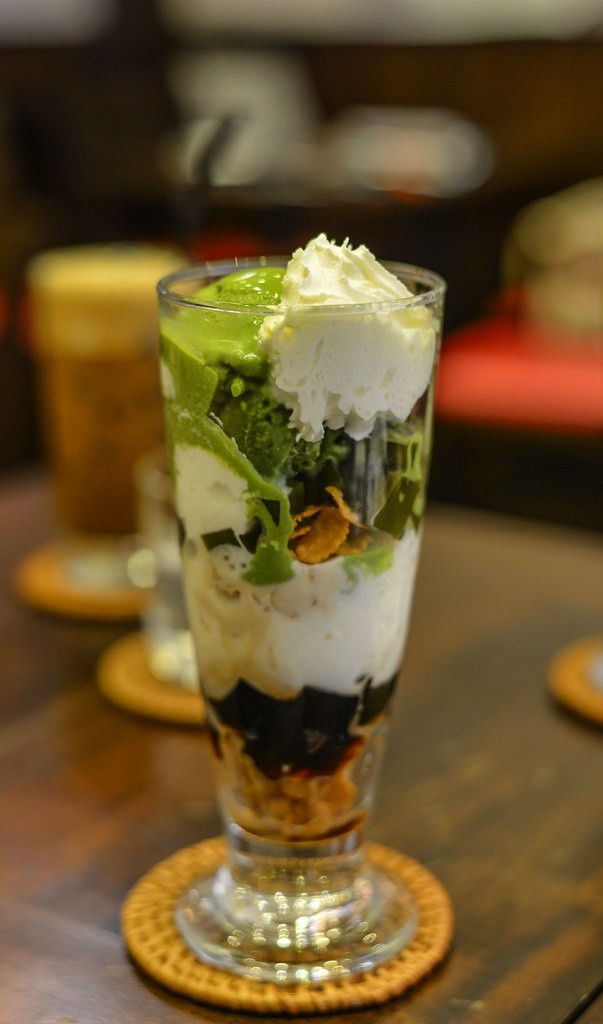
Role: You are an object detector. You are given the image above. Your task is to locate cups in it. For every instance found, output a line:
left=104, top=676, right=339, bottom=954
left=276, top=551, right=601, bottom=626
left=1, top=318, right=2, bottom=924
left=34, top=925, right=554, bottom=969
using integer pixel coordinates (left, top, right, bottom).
left=132, top=443, right=202, bottom=699
left=30, top=247, right=203, bottom=589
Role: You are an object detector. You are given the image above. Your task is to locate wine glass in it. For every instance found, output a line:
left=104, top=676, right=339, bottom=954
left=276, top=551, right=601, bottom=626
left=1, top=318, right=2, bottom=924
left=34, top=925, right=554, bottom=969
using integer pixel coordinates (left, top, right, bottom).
left=157, top=258, right=446, bottom=986
